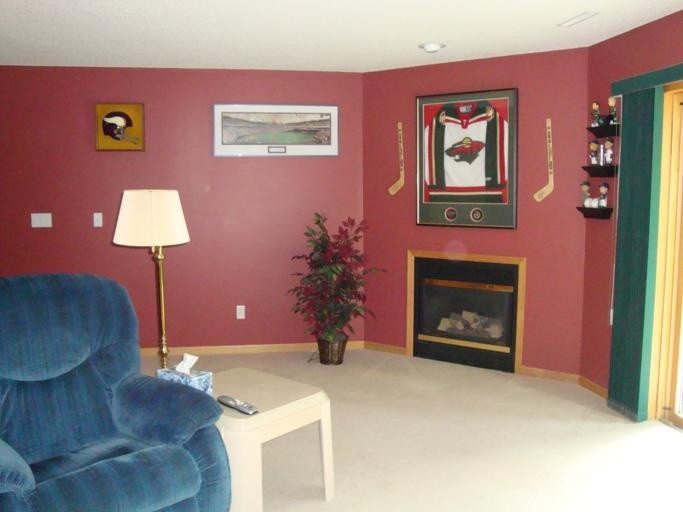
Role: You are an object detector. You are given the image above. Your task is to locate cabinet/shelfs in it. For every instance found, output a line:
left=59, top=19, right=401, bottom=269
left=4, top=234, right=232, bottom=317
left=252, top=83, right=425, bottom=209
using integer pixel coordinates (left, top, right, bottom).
left=572, top=125, right=621, bottom=221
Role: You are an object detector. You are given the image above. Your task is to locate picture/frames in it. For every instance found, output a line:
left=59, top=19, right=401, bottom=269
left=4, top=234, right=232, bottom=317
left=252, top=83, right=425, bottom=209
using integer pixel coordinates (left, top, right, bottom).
left=211, top=100, right=338, bottom=158
left=94, top=101, right=145, bottom=153
left=413, top=87, right=519, bottom=230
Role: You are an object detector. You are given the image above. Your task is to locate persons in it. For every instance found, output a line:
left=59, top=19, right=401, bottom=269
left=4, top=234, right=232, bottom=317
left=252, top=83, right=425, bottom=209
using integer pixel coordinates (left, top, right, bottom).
left=601, top=136, right=613, bottom=166
left=607, top=97, right=617, bottom=124
left=579, top=181, right=592, bottom=208
left=590, top=101, right=600, bottom=127
left=588, top=140, right=599, bottom=165
left=597, top=182, right=609, bottom=208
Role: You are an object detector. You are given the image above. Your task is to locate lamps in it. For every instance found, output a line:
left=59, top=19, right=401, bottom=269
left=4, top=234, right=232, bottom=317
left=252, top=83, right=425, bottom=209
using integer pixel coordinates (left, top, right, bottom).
left=113, top=188, right=190, bottom=368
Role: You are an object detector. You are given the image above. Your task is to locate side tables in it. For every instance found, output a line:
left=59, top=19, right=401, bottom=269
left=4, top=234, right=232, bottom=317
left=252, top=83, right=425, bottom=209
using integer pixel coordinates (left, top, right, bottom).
left=203, top=365, right=336, bottom=512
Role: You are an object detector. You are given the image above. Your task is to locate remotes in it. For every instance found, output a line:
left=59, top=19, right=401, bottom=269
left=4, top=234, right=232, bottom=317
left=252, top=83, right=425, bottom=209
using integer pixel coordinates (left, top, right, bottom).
left=218, top=395, right=259, bottom=415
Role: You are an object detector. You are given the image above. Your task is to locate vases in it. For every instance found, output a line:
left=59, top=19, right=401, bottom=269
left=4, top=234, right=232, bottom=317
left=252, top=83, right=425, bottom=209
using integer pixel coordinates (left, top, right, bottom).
left=315, top=335, right=347, bottom=366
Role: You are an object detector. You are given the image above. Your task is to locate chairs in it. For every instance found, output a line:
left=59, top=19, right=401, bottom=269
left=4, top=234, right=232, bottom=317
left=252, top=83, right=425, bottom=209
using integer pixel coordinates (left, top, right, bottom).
left=0, top=271, right=238, bottom=512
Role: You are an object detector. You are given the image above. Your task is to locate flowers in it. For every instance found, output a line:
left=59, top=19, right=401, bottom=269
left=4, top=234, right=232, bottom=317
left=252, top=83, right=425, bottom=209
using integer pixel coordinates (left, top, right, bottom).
left=284, top=209, right=389, bottom=343
